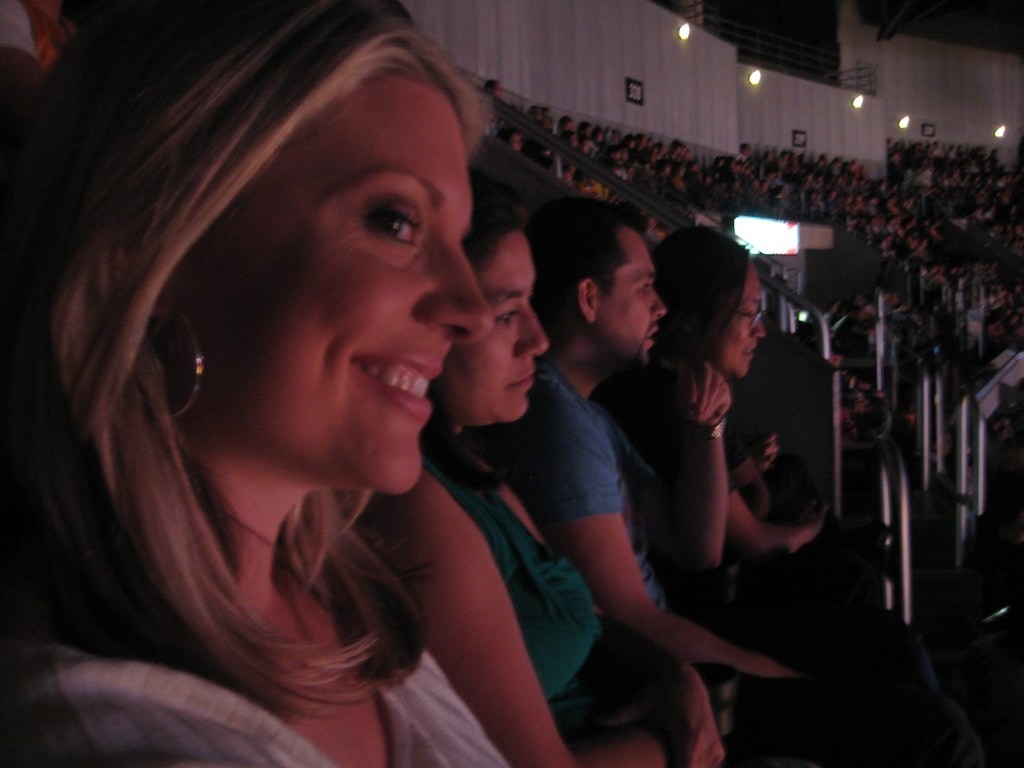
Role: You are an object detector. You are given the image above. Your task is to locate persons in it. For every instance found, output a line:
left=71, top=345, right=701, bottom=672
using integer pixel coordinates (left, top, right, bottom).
left=480, top=71, right=1023, bottom=353
left=1, top=1, right=514, bottom=767
left=357, top=200, right=996, bottom=767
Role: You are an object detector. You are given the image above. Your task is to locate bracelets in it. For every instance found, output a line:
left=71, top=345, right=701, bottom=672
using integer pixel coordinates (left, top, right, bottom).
left=678, top=420, right=728, bottom=442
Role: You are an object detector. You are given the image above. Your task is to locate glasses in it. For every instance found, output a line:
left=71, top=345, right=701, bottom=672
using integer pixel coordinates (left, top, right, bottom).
left=734, top=303, right=768, bottom=326
